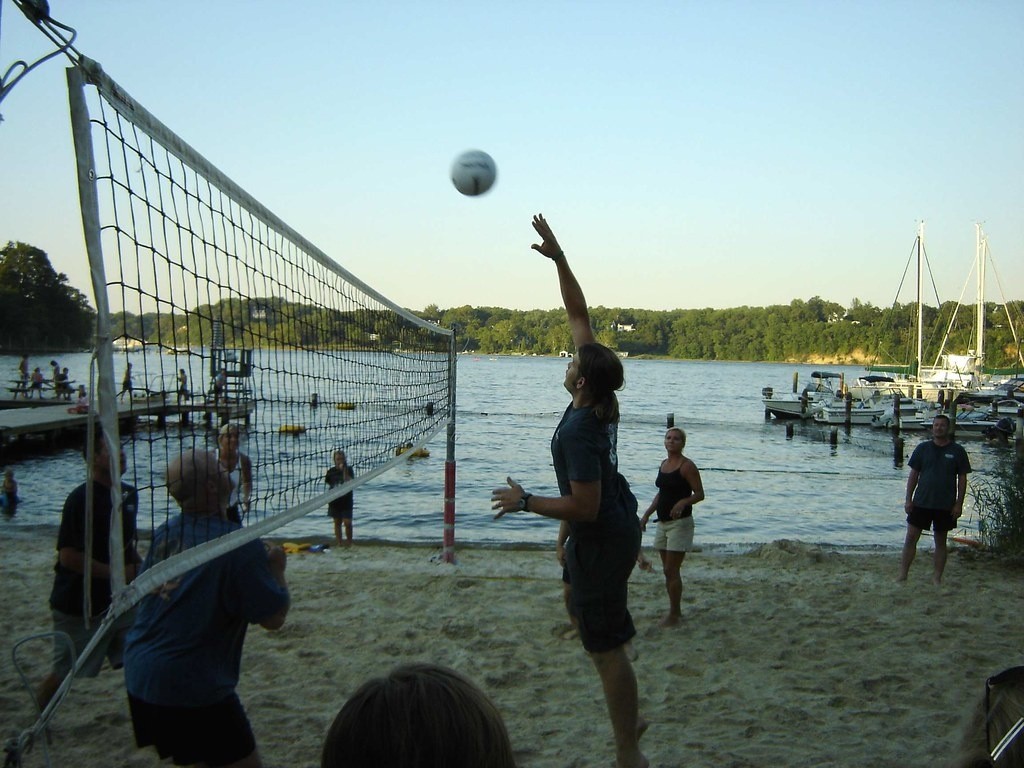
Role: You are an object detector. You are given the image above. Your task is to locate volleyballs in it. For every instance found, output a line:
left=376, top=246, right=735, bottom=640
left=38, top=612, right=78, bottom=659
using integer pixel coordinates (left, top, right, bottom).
left=450, top=147, right=496, bottom=196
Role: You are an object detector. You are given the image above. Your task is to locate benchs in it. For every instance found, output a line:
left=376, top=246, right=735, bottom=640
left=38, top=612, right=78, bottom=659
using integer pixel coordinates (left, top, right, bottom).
left=7, top=387, right=79, bottom=399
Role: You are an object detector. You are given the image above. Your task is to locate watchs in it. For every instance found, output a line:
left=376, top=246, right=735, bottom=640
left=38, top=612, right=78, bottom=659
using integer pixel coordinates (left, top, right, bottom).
left=519, top=492, right=534, bottom=513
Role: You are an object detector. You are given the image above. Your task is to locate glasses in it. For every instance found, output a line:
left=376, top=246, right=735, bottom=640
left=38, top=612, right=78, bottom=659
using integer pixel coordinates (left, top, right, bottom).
left=985, top=665, right=1024, bottom=753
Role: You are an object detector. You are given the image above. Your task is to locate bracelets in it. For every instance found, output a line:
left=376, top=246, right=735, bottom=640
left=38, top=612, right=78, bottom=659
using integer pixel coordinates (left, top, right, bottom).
left=552, top=251, right=564, bottom=261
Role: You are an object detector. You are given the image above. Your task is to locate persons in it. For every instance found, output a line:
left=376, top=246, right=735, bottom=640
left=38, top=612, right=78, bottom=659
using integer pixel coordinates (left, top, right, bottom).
left=206, top=422, right=251, bottom=529
left=1, top=470, right=17, bottom=517
left=640, top=428, right=705, bottom=630
left=124, top=448, right=291, bottom=768
left=324, top=450, right=354, bottom=546
left=491, top=213, right=652, bottom=768
left=29, top=432, right=144, bottom=745
left=938, top=385, right=945, bottom=409
left=16, top=352, right=225, bottom=409
left=320, top=660, right=516, bottom=768
left=893, top=415, right=972, bottom=587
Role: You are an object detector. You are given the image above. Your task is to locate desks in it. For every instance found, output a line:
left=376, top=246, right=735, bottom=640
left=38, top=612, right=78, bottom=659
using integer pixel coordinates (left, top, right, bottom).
left=9, top=380, right=54, bottom=399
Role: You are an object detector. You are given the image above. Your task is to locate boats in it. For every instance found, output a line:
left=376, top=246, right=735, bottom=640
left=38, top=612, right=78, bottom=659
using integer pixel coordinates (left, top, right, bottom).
left=760, top=219, right=1024, bottom=447
